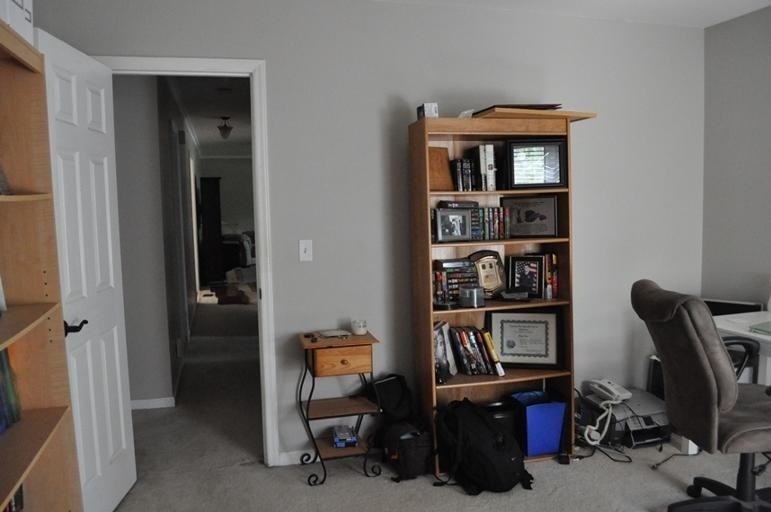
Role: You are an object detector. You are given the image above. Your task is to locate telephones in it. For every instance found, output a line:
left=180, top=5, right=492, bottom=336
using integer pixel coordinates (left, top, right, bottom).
left=590, top=378, right=632, bottom=401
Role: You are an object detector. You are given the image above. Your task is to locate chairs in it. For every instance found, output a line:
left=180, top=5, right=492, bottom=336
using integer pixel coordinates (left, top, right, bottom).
left=239, top=234, right=256, bottom=267
left=630, top=279, right=771, bottom=512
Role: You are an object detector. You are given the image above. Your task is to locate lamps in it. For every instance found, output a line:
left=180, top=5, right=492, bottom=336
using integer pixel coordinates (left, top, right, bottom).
left=217, top=117, right=234, bottom=139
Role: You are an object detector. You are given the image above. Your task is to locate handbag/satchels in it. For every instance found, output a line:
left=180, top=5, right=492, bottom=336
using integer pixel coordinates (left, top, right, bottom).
left=365, top=374, right=414, bottom=424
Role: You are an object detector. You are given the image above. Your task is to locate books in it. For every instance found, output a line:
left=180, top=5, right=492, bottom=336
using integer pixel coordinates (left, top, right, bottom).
left=0, top=276, right=26, bottom=511
left=431, top=200, right=559, bottom=385
left=749, top=320, right=771, bottom=336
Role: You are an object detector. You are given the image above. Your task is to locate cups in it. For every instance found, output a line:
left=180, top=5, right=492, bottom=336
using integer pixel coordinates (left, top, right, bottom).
left=457, top=283, right=487, bottom=309
left=350, top=320, right=368, bottom=336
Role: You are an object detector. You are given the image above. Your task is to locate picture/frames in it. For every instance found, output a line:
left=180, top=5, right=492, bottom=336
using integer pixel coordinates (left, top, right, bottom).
left=484, top=309, right=566, bottom=370
left=505, top=137, right=567, bottom=190
left=499, top=195, right=560, bottom=240
left=433, top=208, right=471, bottom=244
left=508, top=255, right=545, bottom=302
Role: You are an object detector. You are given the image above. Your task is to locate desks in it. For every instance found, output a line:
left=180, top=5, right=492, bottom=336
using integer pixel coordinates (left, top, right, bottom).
left=712, top=311, right=770, bottom=385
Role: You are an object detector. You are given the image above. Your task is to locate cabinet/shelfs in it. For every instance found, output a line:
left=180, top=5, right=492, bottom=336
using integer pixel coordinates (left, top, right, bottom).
left=407, top=117, right=576, bottom=478
left=299, top=328, right=385, bottom=486
left=0, top=19, right=86, bottom=511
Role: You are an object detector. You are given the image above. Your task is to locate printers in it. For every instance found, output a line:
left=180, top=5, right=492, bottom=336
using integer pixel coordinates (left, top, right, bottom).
left=574, top=386, right=669, bottom=448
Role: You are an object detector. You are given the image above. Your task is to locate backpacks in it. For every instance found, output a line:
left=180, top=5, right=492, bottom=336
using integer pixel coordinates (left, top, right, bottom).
left=435, top=398, right=535, bottom=497
left=383, top=417, right=432, bottom=480
